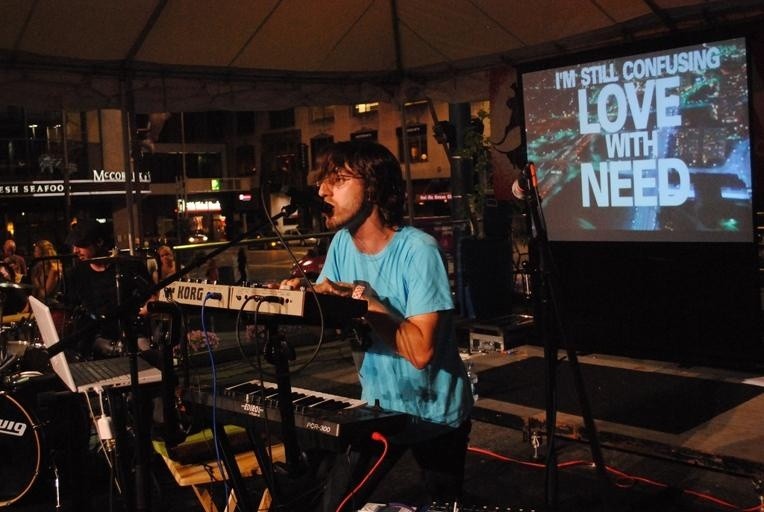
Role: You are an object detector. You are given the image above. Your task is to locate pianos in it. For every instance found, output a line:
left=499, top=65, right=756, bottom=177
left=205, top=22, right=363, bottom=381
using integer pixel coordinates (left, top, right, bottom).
left=181, top=375, right=410, bottom=439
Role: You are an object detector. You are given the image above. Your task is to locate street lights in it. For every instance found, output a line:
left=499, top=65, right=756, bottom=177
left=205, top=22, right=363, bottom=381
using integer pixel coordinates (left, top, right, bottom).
left=29, top=124, right=39, bottom=137
left=53, top=124, right=62, bottom=138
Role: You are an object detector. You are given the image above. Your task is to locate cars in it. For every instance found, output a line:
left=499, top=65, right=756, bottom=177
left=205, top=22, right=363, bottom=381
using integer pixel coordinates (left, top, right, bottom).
left=284, top=229, right=320, bottom=247
left=246, top=229, right=289, bottom=250
left=289, top=225, right=457, bottom=285
left=186, top=233, right=209, bottom=244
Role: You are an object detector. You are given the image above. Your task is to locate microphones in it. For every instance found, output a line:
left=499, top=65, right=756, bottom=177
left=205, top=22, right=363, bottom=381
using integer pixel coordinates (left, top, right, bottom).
left=269, top=175, right=335, bottom=218
left=511, top=162, right=535, bottom=201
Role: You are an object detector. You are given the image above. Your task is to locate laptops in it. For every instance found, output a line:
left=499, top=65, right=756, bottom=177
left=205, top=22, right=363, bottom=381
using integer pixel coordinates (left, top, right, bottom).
left=28, top=294, right=163, bottom=394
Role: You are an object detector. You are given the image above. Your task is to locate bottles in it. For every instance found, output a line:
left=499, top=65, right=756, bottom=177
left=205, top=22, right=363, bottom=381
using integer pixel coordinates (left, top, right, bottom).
left=464, top=361, right=478, bottom=404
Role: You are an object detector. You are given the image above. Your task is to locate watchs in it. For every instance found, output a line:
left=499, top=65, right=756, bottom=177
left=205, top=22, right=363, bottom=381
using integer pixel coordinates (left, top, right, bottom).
left=351, top=280, right=370, bottom=301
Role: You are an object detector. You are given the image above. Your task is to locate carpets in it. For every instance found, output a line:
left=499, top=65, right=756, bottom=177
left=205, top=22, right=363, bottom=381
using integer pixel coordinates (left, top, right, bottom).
left=465, top=354, right=764, bottom=434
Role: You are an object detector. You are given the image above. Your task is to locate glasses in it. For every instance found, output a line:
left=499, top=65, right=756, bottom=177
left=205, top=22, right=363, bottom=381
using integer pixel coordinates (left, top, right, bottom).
left=317, top=175, right=364, bottom=185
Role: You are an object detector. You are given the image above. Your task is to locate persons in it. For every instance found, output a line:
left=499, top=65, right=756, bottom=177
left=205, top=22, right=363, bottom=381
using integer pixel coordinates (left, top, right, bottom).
left=279, top=141, right=474, bottom=512
left=0, top=218, right=219, bottom=359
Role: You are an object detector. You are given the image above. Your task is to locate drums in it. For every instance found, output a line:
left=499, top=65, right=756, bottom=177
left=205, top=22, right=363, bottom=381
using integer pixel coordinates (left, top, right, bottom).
left=0, top=371, right=60, bottom=508
left=0, top=341, right=43, bottom=376
